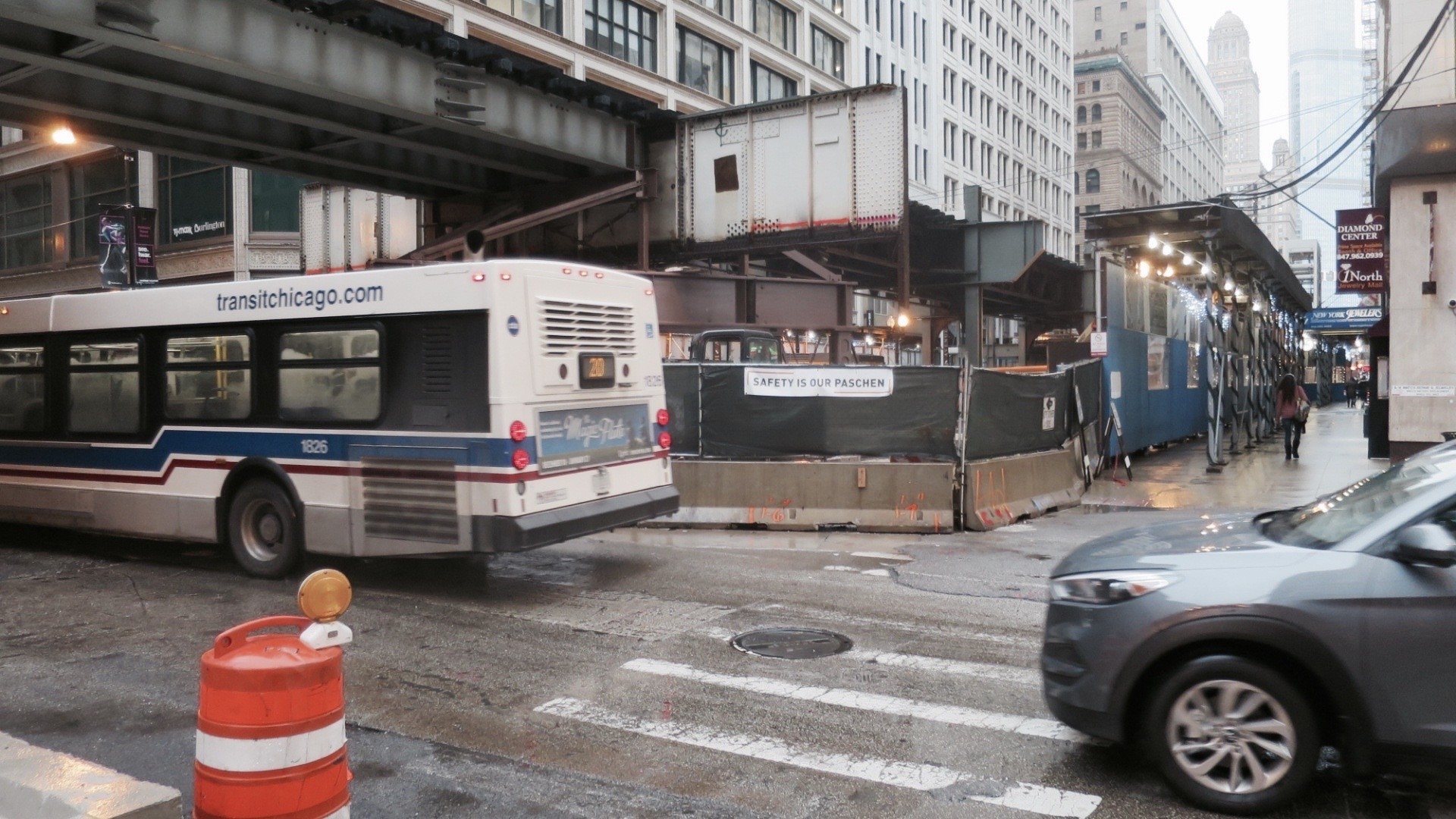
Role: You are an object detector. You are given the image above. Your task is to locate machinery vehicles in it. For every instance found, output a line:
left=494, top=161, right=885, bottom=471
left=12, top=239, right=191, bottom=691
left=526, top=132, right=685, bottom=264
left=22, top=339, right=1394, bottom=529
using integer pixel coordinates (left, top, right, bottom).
left=664, top=328, right=782, bottom=365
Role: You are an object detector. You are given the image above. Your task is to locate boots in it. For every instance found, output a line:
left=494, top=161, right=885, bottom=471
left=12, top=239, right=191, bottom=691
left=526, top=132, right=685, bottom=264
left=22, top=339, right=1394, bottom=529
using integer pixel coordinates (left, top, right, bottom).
left=1283, top=445, right=1290, bottom=459
left=1291, top=439, right=1300, bottom=458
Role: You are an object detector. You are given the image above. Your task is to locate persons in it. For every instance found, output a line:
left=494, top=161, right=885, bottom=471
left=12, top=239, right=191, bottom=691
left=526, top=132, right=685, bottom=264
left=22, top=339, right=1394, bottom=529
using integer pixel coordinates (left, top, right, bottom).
left=1357, top=376, right=1368, bottom=408
left=1274, top=373, right=1310, bottom=459
left=1352, top=378, right=1359, bottom=406
left=1345, top=377, right=1357, bottom=408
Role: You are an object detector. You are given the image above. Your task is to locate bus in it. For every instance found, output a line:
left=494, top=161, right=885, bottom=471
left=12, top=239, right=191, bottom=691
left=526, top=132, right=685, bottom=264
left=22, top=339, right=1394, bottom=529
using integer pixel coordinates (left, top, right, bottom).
left=0, top=257, right=679, bottom=582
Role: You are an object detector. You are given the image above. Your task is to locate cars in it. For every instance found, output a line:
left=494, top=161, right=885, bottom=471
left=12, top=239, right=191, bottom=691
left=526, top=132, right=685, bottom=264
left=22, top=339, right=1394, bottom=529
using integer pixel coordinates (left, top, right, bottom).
left=1038, top=435, right=1456, bottom=814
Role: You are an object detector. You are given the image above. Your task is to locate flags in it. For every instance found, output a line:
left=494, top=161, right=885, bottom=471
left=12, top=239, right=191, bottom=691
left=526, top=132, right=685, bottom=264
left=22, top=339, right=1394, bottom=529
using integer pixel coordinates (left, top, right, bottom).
left=131, top=206, right=158, bottom=284
left=99, top=202, right=131, bottom=289
